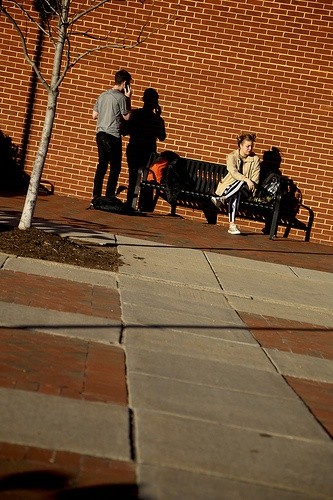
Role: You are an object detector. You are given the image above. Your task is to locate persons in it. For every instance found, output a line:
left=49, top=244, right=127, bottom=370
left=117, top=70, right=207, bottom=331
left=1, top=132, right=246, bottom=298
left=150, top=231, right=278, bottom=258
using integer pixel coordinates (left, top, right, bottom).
left=87, top=68, right=133, bottom=210
left=208, top=131, right=262, bottom=235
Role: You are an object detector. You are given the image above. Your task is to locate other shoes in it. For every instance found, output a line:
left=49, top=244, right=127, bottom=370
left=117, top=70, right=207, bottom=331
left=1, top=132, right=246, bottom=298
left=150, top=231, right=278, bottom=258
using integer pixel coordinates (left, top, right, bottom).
left=103, top=198, right=136, bottom=215
left=90, top=197, right=107, bottom=210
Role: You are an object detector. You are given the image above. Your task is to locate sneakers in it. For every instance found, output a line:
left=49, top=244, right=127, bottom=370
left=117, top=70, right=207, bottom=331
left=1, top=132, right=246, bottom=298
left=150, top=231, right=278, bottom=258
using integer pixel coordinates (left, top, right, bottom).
left=228, top=225, right=241, bottom=234
left=211, top=197, right=226, bottom=211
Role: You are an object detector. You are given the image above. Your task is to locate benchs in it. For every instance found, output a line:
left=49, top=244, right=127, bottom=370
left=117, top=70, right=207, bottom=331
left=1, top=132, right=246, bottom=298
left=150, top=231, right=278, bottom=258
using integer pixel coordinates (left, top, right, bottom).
left=150, top=151, right=283, bottom=241
